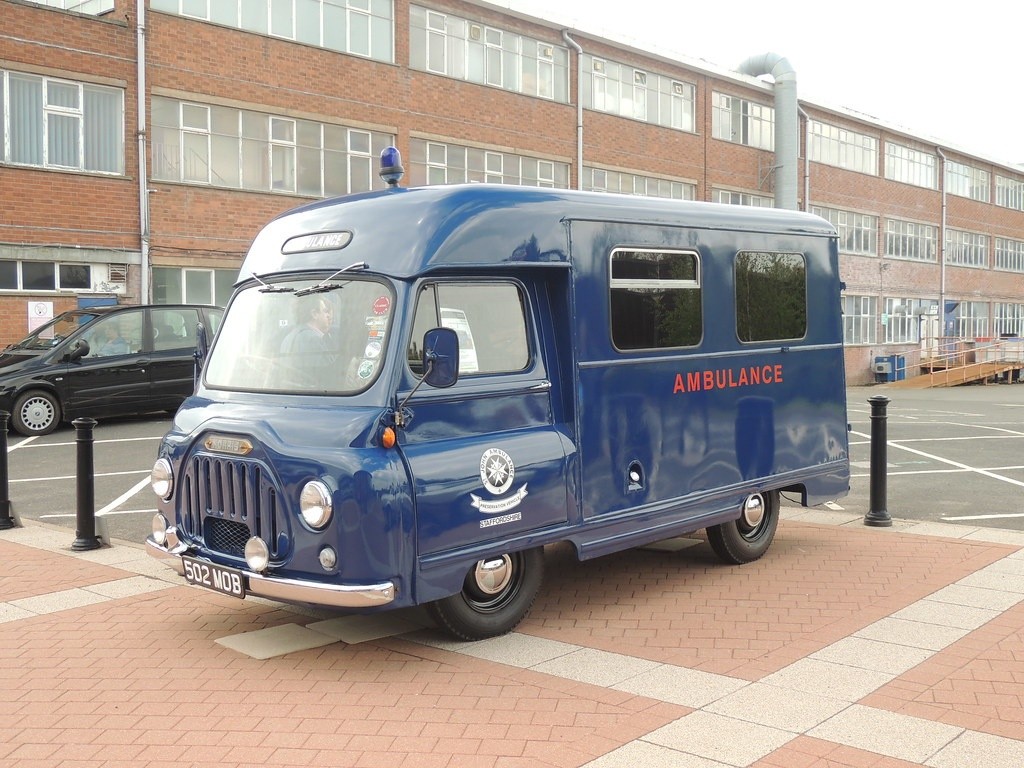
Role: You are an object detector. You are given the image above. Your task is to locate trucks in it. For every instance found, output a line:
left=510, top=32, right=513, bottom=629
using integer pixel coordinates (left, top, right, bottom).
left=145, top=146, right=847, bottom=642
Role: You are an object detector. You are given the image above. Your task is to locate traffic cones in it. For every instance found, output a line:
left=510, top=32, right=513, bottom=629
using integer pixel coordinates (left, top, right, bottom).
left=863, top=395, right=893, bottom=527
left=0, top=405, right=15, bottom=531
left=71, top=415, right=101, bottom=552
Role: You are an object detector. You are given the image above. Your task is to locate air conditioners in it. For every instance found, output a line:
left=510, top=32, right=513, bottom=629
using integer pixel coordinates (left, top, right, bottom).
left=875, top=361, right=892, bottom=373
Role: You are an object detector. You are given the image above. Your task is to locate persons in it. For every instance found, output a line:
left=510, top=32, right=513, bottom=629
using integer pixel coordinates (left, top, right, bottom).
left=93, top=321, right=130, bottom=356
left=283, top=298, right=339, bottom=380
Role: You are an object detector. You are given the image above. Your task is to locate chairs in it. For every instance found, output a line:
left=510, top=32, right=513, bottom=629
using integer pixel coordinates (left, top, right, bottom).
left=159, top=325, right=179, bottom=344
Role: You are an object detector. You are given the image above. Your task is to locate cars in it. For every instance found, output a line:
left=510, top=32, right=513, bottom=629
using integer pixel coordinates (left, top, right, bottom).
left=1, top=308, right=227, bottom=437
